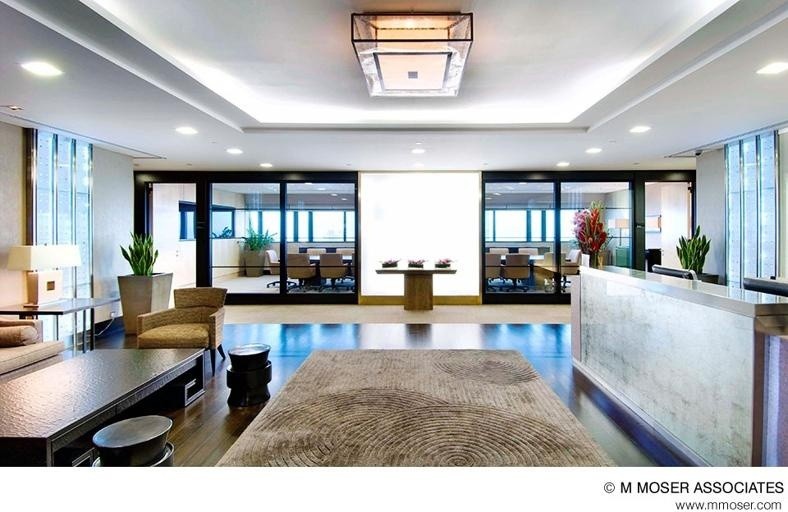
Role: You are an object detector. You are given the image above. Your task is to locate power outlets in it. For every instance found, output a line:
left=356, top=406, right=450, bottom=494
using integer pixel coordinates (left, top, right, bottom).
left=110, top=311, right=115, bottom=319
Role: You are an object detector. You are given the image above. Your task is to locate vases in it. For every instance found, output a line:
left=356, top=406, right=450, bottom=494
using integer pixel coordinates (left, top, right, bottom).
left=434, top=264, right=449, bottom=268
left=381, top=264, right=397, bottom=267
left=582, top=252, right=600, bottom=268
left=407, top=264, right=422, bottom=268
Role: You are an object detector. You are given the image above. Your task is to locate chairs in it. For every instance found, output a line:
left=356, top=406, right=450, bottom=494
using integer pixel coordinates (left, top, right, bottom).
left=518, top=248, right=538, bottom=257
left=286, top=253, right=316, bottom=292
left=306, top=248, right=325, bottom=256
left=533, top=252, right=565, bottom=293
left=264, top=249, right=295, bottom=288
left=350, top=252, right=355, bottom=293
left=318, top=254, right=348, bottom=292
left=485, top=253, right=502, bottom=292
left=562, top=249, right=581, bottom=292
left=489, top=248, right=508, bottom=255
left=335, top=249, right=354, bottom=256
left=499, top=254, right=531, bottom=293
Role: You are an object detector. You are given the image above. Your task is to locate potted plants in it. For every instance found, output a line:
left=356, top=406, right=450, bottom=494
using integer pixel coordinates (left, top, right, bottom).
left=239, top=226, right=276, bottom=277
left=675, top=224, right=720, bottom=285
left=117, top=230, right=174, bottom=337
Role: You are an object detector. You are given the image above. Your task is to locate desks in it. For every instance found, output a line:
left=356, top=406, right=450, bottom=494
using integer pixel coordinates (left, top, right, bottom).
left=499, top=254, right=571, bottom=288
left=375, top=267, right=457, bottom=312
left=0, top=298, right=121, bottom=354
left=644, top=248, right=662, bottom=274
left=277, top=254, right=356, bottom=286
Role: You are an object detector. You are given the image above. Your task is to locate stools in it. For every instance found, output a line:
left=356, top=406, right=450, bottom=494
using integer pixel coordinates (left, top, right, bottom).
left=90, top=415, right=175, bottom=467
left=224, top=342, right=271, bottom=407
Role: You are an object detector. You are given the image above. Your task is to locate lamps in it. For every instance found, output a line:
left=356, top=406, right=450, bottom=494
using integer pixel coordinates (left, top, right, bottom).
left=349, top=12, right=474, bottom=100
left=607, top=217, right=630, bottom=247
left=5, top=244, right=82, bottom=307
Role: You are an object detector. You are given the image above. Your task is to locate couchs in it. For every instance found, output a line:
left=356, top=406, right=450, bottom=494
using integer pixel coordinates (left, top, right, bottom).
left=0, top=317, right=65, bottom=386
left=135, top=286, right=227, bottom=376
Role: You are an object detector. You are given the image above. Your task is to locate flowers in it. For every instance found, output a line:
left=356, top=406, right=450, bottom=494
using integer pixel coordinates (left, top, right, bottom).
left=383, top=258, right=399, bottom=264
left=406, top=259, right=425, bottom=264
left=570, top=198, right=613, bottom=267
left=437, top=259, right=452, bottom=264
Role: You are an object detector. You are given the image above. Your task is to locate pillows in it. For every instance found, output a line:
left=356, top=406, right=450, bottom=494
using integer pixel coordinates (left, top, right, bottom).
left=0, top=326, right=38, bottom=346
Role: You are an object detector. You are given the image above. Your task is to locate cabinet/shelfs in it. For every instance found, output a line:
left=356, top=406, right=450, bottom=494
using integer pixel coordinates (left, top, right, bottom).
left=615, top=246, right=631, bottom=267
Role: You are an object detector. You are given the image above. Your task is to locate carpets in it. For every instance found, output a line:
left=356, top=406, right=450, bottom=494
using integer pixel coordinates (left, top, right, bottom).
left=214, top=347, right=618, bottom=466
